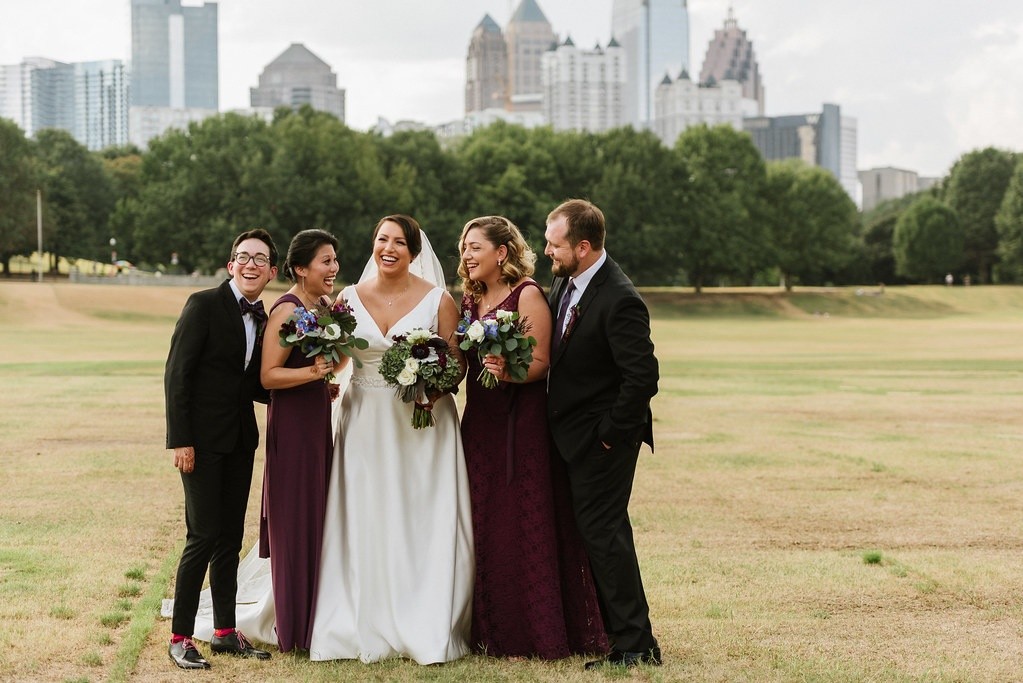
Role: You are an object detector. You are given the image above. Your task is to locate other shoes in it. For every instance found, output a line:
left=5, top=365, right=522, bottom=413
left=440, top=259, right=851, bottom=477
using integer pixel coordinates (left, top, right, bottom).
left=584, top=646, right=661, bottom=670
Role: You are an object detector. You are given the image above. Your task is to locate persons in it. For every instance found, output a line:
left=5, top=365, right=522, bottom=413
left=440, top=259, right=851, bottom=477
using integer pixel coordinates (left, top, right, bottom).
left=543, top=199, right=665, bottom=672
left=455, top=215, right=610, bottom=661
left=259, top=230, right=351, bottom=656
left=322, top=214, right=471, bottom=666
left=163, top=228, right=279, bottom=671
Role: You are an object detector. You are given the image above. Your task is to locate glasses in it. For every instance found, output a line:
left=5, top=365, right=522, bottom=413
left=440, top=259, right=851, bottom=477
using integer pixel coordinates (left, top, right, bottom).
left=234, top=251, right=271, bottom=268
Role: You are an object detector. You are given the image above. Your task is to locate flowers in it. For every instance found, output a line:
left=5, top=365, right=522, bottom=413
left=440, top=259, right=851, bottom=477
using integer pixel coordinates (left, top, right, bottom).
left=279, top=297, right=366, bottom=382
left=457, top=310, right=536, bottom=386
left=377, top=325, right=461, bottom=431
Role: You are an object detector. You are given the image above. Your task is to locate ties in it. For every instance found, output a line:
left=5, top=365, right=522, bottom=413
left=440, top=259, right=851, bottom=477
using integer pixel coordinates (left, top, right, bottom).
left=554, top=277, right=577, bottom=354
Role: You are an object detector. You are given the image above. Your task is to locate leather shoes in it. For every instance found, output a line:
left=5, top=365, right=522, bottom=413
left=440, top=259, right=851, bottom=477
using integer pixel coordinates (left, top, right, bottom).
left=168, top=638, right=211, bottom=669
left=210, top=631, right=272, bottom=660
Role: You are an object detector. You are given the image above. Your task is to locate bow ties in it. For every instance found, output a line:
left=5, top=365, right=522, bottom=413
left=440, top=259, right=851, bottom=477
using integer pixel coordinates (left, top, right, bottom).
left=239, top=297, right=265, bottom=321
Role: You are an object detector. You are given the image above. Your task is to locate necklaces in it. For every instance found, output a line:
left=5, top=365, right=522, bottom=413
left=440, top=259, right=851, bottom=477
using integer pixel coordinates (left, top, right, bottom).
left=481, top=284, right=506, bottom=310
left=375, top=272, right=410, bottom=308
